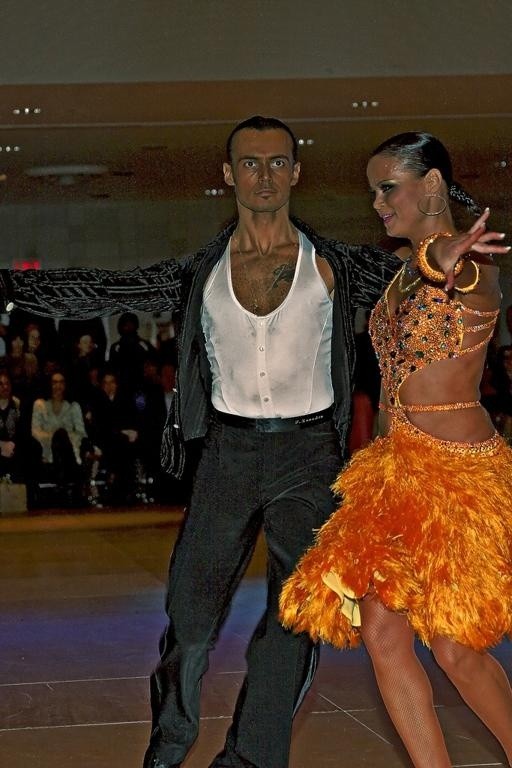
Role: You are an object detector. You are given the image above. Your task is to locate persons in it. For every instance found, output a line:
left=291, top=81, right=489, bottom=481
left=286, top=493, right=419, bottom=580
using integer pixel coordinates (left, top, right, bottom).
left=484, top=345, right=512, bottom=411
left=1, top=115, right=406, bottom=768
left=2, top=310, right=185, bottom=513
left=354, top=309, right=380, bottom=408
left=279, top=131, right=512, bottom=768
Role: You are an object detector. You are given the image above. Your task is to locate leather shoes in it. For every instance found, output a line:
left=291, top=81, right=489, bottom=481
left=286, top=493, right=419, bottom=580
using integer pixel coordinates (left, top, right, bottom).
left=143, top=746, right=181, bottom=768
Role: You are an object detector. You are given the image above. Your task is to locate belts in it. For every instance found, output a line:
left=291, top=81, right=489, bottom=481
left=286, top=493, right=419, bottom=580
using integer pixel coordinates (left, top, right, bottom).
left=216, top=403, right=336, bottom=432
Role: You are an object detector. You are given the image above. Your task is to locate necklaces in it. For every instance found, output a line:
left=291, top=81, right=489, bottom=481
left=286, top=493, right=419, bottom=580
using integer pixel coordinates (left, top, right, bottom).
left=229, top=233, right=299, bottom=313
left=393, top=264, right=423, bottom=292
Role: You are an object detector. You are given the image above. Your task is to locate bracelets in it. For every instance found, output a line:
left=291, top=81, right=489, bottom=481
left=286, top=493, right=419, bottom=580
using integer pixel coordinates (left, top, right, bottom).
left=419, top=231, right=463, bottom=283
left=455, top=257, right=481, bottom=292
